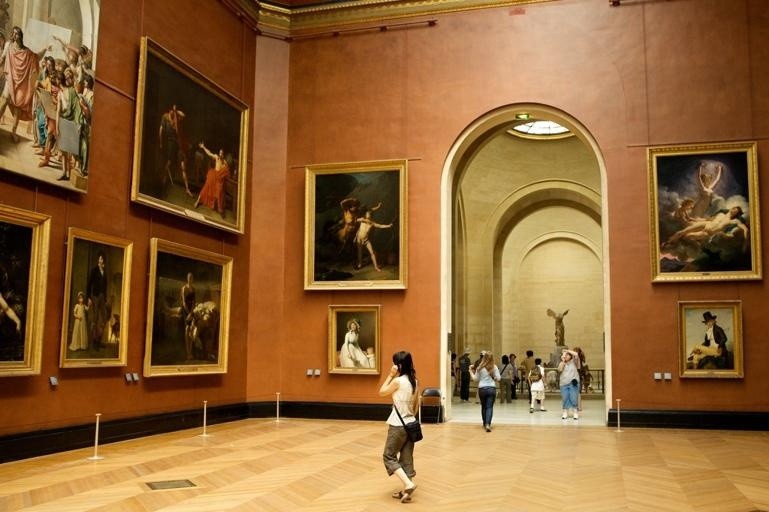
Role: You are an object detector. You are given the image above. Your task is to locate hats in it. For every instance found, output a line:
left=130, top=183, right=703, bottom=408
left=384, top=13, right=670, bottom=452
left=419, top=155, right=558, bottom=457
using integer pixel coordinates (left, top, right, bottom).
left=347, top=318, right=361, bottom=334
left=701, top=311, right=717, bottom=323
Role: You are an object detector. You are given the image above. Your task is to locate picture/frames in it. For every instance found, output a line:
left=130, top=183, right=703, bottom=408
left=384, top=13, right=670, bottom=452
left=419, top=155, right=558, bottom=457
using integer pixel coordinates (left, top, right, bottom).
left=140, top=236, right=234, bottom=380
left=673, top=297, right=745, bottom=383
left=130, top=32, right=251, bottom=239
left=56, top=223, right=135, bottom=372
left=0, top=202, right=55, bottom=381
left=302, top=158, right=412, bottom=293
left=326, top=302, right=384, bottom=376
left=642, top=140, right=767, bottom=287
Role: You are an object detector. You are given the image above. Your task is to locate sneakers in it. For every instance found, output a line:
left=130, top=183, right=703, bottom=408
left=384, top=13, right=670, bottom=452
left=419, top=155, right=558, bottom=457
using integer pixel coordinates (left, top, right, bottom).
left=561, top=413, right=568, bottom=419
left=572, top=411, right=579, bottom=419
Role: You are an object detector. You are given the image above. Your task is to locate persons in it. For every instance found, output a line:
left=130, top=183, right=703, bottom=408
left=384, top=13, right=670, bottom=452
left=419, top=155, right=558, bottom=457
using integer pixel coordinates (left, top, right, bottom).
left=335, top=197, right=383, bottom=261
left=363, top=345, right=376, bottom=368
left=66, top=291, right=92, bottom=351
left=192, top=138, right=232, bottom=219
left=687, top=311, right=732, bottom=369
left=0, top=24, right=92, bottom=188
left=181, top=271, right=197, bottom=360
left=159, top=102, right=194, bottom=199
left=671, top=198, right=703, bottom=224
left=1, top=291, right=23, bottom=332
left=693, top=161, right=725, bottom=215
left=558, top=350, right=581, bottom=419
left=469, top=351, right=501, bottom=432
left=377, top=350, right=422, bottom=503
left=87, top=249, right=110, bottom=344
left=450, top=346, right=593, bottom=405
left=339, top=317, right=370, bottom=367
left=661, top=207, right=748, bottom=257
left=353, top=210, right=392, bottom=273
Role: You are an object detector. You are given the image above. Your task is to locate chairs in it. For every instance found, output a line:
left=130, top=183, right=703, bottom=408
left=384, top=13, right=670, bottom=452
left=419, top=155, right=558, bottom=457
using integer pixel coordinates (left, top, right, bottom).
left=415, top=387, right=443, bottom=425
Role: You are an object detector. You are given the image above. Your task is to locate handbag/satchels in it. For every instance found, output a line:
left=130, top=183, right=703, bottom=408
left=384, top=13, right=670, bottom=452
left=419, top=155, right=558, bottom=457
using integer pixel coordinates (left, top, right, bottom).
left=403, top=421, right=424, bottom=444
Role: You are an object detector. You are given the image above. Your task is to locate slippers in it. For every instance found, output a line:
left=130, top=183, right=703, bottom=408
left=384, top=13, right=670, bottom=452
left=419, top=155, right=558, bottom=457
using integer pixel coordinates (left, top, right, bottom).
left=391, top=480, right=419, bottom=505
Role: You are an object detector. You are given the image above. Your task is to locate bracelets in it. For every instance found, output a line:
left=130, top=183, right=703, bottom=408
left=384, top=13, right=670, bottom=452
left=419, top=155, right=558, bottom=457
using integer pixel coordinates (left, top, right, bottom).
left=388, top=374, right=394, bottom=378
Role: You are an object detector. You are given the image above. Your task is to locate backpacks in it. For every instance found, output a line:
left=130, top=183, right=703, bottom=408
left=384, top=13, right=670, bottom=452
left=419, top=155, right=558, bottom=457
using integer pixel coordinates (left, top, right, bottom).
left=529, top=365, right=542, bottom=383
